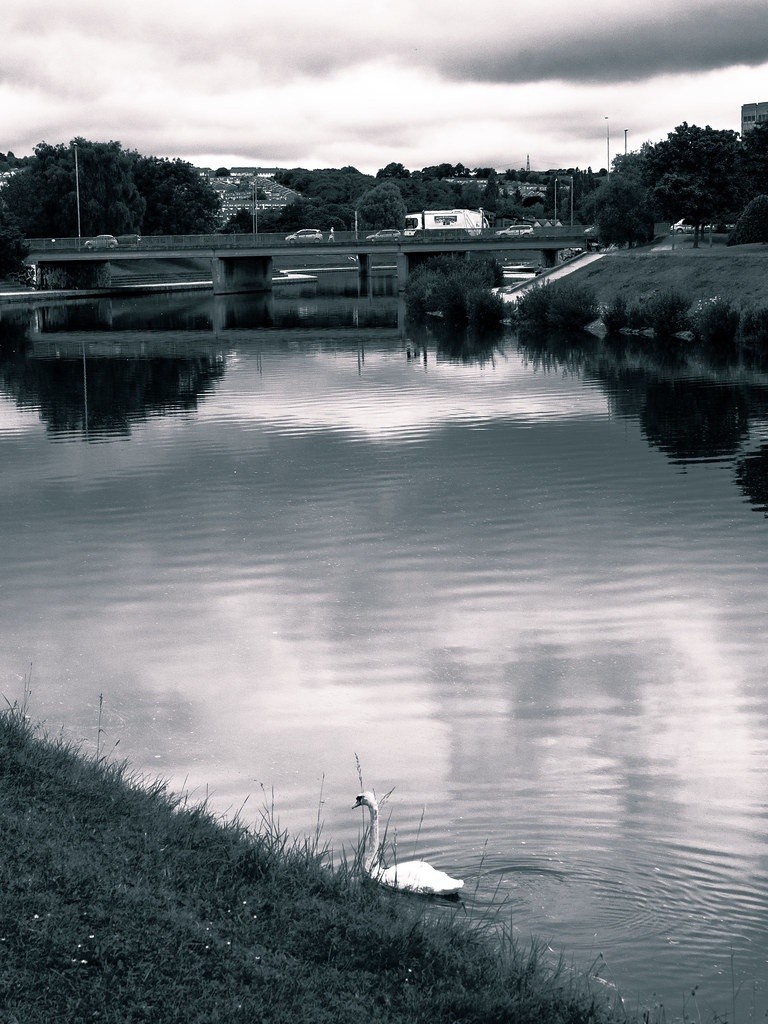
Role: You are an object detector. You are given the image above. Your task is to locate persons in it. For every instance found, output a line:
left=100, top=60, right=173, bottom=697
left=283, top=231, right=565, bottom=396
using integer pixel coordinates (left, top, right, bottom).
left=328, top=227, right=335, bottom=242
left=535, top=264, right=544, bottom=276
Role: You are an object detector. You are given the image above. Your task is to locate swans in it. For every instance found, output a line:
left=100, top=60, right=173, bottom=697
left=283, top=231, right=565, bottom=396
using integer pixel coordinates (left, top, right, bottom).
left=351, top=791, right=465, bottom=897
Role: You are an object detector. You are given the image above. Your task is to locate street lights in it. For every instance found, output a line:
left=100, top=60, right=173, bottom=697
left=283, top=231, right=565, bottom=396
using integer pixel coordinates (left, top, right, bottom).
left=73, top=143, right=81, bottom=252
left=570, top=176, right=573, bottom=227
left=605, top=116, right=610, bottom=183
left=555, top=178, right=558, bottom=226
left=480, top=207, right=484, bottom=237
left=624, top=129, right=628, bottom=155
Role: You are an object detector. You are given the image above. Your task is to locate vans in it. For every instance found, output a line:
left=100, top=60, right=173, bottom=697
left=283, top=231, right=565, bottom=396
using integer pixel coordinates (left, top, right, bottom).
left=670, top=218, right=717, bottom=233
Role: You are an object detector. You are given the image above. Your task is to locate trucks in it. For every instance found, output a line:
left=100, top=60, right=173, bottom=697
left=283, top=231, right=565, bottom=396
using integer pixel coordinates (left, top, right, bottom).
left=404, top=209, right=481, bottom=237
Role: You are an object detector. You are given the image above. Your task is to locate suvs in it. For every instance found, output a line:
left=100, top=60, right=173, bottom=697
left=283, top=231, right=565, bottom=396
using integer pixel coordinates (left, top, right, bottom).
left=285, top=229, right=323, bottom=243
left=496, top=225, right=534, bottom=239
left=366, top=229, right=401, bottom=242
left=85, top=235, right=118, bottom=249
